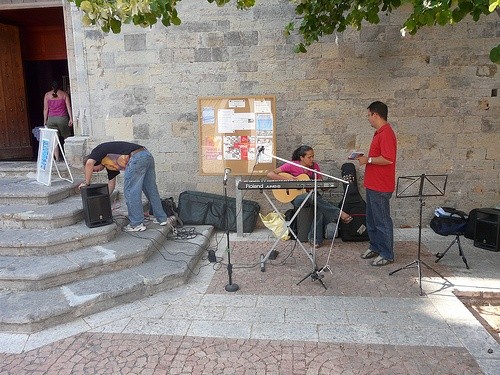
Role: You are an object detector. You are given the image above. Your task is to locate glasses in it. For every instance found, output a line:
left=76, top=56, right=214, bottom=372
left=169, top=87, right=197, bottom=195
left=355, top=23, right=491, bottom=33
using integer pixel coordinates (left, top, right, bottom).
left=366, top=113, right=374, bottom=118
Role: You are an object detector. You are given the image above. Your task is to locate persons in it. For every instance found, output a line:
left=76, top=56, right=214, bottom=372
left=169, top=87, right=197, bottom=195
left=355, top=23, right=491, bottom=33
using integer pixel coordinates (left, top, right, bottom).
left=78, top=141, right=168, bottom=232
left=354, top=102, right=397, bottom=266
left=266, top=144, right=353, bottom=247
left=42, top=72, right=73, bottom=161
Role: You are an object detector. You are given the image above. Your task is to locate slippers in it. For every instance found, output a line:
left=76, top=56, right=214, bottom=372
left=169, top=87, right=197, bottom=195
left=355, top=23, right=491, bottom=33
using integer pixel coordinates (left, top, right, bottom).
left=341, top=216, right=354, bottom=224
left=309, top=240, right=320, bottom=248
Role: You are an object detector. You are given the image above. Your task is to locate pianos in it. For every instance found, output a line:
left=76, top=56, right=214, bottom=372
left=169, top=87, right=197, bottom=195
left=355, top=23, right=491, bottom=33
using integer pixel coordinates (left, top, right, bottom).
left=236, top=178, right=340, bottom=273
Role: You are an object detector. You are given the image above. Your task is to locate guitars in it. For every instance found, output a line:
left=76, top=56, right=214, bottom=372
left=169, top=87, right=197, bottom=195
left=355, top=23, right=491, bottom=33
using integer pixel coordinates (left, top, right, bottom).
left=271, top=172, right=356, bottom=204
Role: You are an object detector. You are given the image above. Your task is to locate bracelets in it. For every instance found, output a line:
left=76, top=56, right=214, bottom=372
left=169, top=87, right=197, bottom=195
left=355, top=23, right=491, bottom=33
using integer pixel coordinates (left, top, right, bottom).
left=84, top=181, right=91, bottom=186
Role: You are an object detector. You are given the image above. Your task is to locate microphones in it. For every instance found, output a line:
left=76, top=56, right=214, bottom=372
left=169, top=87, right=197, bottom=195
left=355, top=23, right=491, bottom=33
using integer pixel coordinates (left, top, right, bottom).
left=223, top=167, right=231, bottom=184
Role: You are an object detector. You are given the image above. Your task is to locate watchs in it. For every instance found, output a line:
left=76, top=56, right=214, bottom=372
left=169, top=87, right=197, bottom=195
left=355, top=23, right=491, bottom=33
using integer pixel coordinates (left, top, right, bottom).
left=368, top=156, right=372, bottom=164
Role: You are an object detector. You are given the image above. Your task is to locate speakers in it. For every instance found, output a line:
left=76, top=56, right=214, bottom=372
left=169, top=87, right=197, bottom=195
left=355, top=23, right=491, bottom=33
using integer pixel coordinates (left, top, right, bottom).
left=473, top=208, right=500, bottom=252
left=80, top=183, right=114, bottom=229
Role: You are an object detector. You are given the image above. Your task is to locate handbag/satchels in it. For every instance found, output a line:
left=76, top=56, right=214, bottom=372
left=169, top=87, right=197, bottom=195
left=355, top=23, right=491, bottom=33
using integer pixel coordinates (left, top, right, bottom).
left=430, top=207, right=468, bottom=236
left=259, top=209, right=291, bottom=241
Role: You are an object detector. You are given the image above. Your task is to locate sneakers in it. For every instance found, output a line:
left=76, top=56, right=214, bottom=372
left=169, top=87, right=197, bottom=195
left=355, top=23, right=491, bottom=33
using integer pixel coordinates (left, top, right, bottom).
left=360, top=249, right=379, bottom=259
left=121, top=223, right=147, bottom=232
left=370, top=255, right=394, bottom=266
left=153, top=218, right=168, bottom=226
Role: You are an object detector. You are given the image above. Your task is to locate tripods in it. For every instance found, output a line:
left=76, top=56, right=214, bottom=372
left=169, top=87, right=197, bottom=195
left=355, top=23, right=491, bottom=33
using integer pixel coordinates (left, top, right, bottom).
left=387, top=174, right=453, bottom=296
